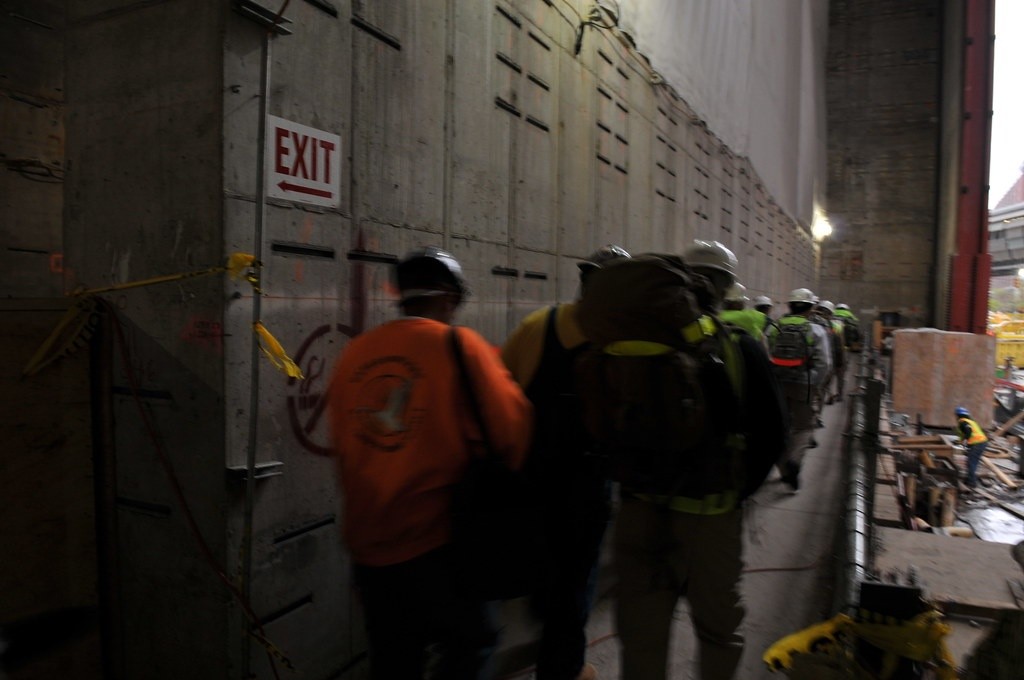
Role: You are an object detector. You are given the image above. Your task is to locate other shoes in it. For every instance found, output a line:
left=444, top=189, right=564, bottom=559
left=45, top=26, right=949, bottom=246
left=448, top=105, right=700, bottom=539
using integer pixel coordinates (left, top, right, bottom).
left=781, top=461, right=799, bottom=490
left=965, top=478, right=976, bottom=488
left=815, top=417, right=823, bottom=429
left=575, top=662, right=597, bottom=680
left=828, top=395, right=834, bottom=404
left=808, top=436, right=817, bottom=449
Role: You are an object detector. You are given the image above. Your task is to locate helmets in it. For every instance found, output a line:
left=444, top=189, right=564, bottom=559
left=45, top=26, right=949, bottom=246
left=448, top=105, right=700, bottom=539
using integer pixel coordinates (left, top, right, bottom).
left=682, top=241, right=737, bottom=279
left=835, top=304, right=850, bottom=310
left=785, top=289, right=820, bottom=304
left=722, top=283, right=750, bottom=302
left=754, top=296, right=772, bottom=308
left=396, top=245, right=466, bottom=299
left=814, top=300, right=836, bottom=315
left=577, top=243, right=632, bottom=272
left=955, top=407, right=970, bottom=415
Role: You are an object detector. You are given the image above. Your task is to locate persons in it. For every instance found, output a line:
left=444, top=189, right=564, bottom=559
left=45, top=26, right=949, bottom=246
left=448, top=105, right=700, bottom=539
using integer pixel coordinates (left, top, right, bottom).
left=501, top=245, right=630, bottom=680
left=726, top=282, right=860, bottom=492
left=954, top=406, right=987, bottom=489
left=327, top=247, right=533, bottom=680
left=611, top=240, right=785, bottom=680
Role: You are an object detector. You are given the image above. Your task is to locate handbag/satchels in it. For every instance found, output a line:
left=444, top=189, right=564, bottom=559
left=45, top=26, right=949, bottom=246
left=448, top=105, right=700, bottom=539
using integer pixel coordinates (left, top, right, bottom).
left=443, top=461, right=537, bottom=598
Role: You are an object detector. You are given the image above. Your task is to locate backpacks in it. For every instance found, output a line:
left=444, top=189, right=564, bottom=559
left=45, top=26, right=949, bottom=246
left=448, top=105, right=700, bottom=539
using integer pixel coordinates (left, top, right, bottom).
left=518, top=307, right=609, bottom=568
left=770, top=321, right=814, bottom=379
left=574, top=252, right=731, bottom=500
